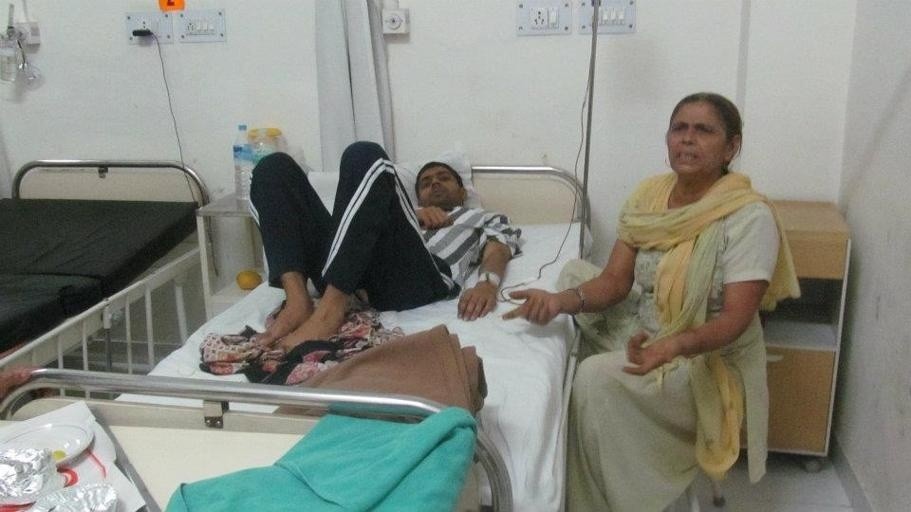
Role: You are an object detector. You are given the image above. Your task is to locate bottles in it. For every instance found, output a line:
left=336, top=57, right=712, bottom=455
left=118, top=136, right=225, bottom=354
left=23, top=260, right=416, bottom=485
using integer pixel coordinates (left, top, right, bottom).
left=233, top=124, right=283, bottom=200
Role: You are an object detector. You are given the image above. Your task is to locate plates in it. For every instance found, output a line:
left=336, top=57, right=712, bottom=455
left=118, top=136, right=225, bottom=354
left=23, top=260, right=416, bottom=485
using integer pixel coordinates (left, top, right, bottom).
left=1, top=422, right=94, bottom=466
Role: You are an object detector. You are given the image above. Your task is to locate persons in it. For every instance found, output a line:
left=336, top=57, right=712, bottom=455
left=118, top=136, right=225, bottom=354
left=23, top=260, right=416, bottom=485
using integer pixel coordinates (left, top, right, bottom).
left=248, top=142, right=525, bottom=352
left=497, top=90, right=788, bottom=509
left=1, top=361, right=37, bottom=404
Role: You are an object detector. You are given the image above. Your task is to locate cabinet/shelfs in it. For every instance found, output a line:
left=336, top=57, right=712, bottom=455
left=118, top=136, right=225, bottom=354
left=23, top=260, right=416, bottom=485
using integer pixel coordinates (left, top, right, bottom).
left=740, top=233, right=851, bottom=456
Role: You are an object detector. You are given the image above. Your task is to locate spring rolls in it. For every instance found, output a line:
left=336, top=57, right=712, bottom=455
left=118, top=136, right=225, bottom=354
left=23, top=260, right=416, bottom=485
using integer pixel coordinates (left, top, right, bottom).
left=77, top=482, right=118, bottom=511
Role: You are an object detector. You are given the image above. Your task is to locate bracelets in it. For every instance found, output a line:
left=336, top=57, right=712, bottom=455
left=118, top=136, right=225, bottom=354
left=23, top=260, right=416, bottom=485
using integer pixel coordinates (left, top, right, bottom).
left=572, top=285, right=588, bottom=314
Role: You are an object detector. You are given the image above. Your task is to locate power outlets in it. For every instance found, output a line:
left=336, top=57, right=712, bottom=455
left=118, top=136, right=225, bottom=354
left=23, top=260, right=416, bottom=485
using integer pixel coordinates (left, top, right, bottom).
left=517, top=0, right=573, bottom=35
left=126, top=12, right=173, bottom=44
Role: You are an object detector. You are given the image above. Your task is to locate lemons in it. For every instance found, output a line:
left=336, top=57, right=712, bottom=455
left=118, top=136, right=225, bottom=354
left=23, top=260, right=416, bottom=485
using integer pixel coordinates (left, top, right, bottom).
left=237, top=270, right=262, bottom=290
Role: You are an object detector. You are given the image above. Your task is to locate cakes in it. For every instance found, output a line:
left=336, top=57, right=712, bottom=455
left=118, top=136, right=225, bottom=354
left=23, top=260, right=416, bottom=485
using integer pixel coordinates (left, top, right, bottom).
left=1, top=444, right=56, bottom=506
